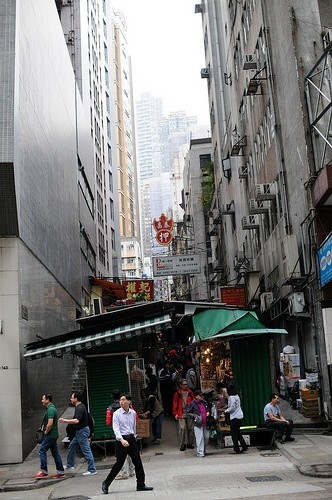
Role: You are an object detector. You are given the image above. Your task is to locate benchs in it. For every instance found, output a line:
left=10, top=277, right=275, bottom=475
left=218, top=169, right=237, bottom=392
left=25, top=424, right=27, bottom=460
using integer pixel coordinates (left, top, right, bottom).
left=216, top=427, right=277, bottom=447
left=90, top=438, right=143, bottom=457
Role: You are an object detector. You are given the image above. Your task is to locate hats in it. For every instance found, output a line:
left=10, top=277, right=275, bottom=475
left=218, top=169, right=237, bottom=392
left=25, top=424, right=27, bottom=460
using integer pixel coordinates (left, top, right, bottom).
left=194, top=390, right=204, bottom=396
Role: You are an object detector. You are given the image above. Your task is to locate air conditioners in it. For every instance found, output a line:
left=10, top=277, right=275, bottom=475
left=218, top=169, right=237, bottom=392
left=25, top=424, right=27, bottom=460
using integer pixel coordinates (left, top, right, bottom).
left=234, top=251, right=244, bottom=269
left=255, top=183, right=275, bottom=200
left=213, top=260, right=221, bottom=271
left=245, top=69, right=258, bottom=93
left=195, top=4, right=202, bottom=13
left=93, top=297, right=104, bottom=314
left=209, top=209, right=220, bottom=232
left=323, top=29, right=332, bottom=51
left=288, top=292, right=305, bottom=313
left=250, top=199, right=258, bottom=209
left=260, top=292, right=273, bottom=311
left=238, top=166, right=248, bottom=178
left=243, top=54, right=256, bottom=69
left=201, top=68, right=209, bottom=78
left=241, top=215, right=259, bottom=228
left=221, top=204, right=234, bottom=215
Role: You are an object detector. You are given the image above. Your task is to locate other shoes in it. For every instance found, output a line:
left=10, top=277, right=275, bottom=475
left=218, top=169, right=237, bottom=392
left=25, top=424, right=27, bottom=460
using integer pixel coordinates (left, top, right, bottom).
left=286, top=437, right=298, bottom=441
left=102, top=482, right=108, bottom=494
left=63, top=466, right=74, bottom=472
left=51, top=473, right=65, bottom=479
left=137, top=485, right=153, bottom=491
left=180, top=444, right=186, bottom=451
left=115, top=476, right=129, bottom=481
left=82, top=471, right=97, bottom=476
left=196, top=451, right=207, bottom=457
left=279, top=439, right=284, bottom=444
left=241, top=448, right=249, bottom=453
left=229, top=450, right=242, bottom=454
left=187, top=444, right=194, bottom=449
left=35, top=471, right=48, bottom=479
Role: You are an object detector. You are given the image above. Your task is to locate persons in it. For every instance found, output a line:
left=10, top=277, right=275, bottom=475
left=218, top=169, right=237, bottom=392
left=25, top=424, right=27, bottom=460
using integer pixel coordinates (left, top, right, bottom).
left=217, top=385, right=249, bottom=454
left=151, top=337, right=194, bottom=419
left=106, top=389, right=139, bottom=480
left=263, top=393, right=295, bottom=444
left=34, top=393, right=65, bottom=479
left=59, top=391, right=97, bottom=476
left=172, top=379, right=195, bottom=451
left=140, top=389, right=164, bottom=445
left=102, top=393, right=153, bottom=493
left=186, top=390, right=210, bottom=458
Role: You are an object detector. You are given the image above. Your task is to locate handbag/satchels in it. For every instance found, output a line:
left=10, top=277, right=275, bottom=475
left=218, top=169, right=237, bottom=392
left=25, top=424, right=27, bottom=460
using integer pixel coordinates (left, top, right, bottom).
left=35, top=430, right=44, bottom=444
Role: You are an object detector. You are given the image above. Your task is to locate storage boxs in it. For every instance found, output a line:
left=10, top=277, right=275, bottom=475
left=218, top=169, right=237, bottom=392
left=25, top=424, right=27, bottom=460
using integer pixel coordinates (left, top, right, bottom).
left=137, top=419, right=152, bottom=438
left=225, top=435, right=250, bottom=447
left=279, top=352, right=320, bottom=417
